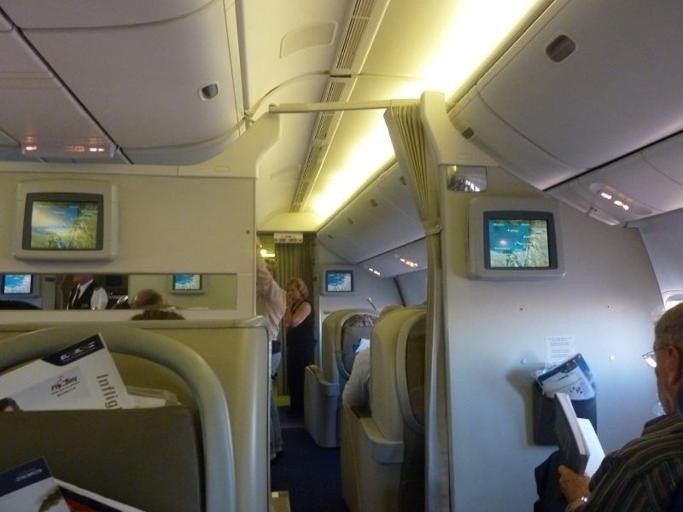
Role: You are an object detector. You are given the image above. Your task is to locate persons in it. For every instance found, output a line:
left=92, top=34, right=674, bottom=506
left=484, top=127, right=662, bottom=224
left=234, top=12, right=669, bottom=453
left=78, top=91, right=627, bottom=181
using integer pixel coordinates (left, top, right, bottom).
left=111, top=289, right=185, bottom=320
left=284, top=277, right=314, bottom=419
left=256, top=235, right=288, bottom=460
left=558, top=302, right=683, bottom=512
left=342, top=305, right=404, bottom=408
left=66, top=273, right=109, bottom=310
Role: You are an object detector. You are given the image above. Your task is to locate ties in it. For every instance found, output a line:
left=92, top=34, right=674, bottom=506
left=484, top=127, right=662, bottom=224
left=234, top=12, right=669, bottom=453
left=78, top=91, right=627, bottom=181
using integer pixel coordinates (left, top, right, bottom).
left=71, top=285, right=81, bottom=305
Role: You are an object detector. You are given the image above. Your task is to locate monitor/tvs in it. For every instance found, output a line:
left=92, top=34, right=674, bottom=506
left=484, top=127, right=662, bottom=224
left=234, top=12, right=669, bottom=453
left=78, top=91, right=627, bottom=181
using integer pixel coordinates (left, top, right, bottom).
left=325, top=269, right=354, bottom=292
left=13, top=181, right=116, bottom=259
left=468, top=196, right=569, bottom=278
left=172, top=274, right=206, bottom=292
left=2, top=275, right=39, bottom=294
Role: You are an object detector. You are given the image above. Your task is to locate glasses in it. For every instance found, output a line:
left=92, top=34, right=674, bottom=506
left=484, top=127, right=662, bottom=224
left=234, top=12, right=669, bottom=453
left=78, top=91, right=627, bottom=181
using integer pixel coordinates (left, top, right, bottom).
left=641, top=345, right=670, bottom=369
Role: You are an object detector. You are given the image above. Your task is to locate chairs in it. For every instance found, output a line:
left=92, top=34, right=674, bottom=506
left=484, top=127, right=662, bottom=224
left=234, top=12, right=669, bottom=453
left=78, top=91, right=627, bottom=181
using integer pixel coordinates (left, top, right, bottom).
left=306, top=302, right=431, bottom=511
left=0, top=298, right=292, bottom=512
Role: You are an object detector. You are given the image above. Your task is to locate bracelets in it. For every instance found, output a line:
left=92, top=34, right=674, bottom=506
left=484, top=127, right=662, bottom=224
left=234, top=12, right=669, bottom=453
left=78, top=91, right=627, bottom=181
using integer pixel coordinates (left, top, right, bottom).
left=565, top=496, right=588, bottom=512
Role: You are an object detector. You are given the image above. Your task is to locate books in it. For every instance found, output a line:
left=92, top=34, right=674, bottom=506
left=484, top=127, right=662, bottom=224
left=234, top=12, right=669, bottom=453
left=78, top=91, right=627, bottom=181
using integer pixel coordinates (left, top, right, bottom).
left=552, top=392, right=606, bottom=479
left=54, top=478, right=146, bottom=512
left=126, top=385, right=183, bottom=409
left=0, top=331, right=179, bottom=412
left=0, top=456, right=71, bottom=512
